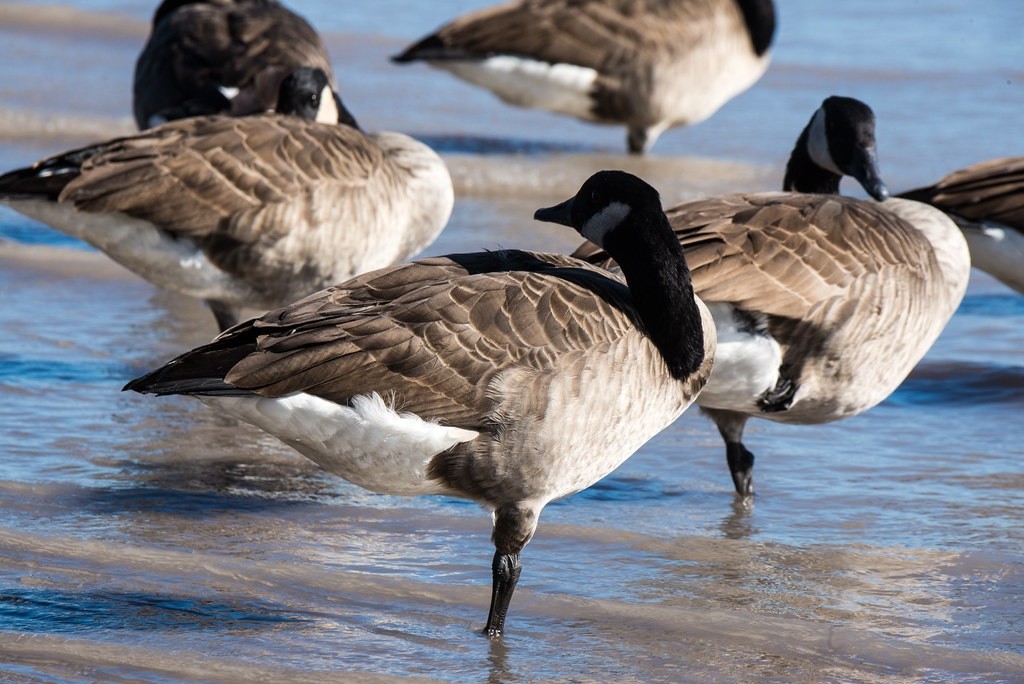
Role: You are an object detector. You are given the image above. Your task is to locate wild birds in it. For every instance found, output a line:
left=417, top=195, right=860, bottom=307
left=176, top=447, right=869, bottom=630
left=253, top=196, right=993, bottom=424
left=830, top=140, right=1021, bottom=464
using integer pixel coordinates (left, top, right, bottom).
left=132, top=1, right=324, bottom=135
left=892, top=158, right=1024, bottom=298
left=568, top=93, right=973, bottom=497
left=120, top=168, right=717, bottom=638
left=386, top=1, right=779, bottom=156
left=1, top=66, right=455, bottom=333
